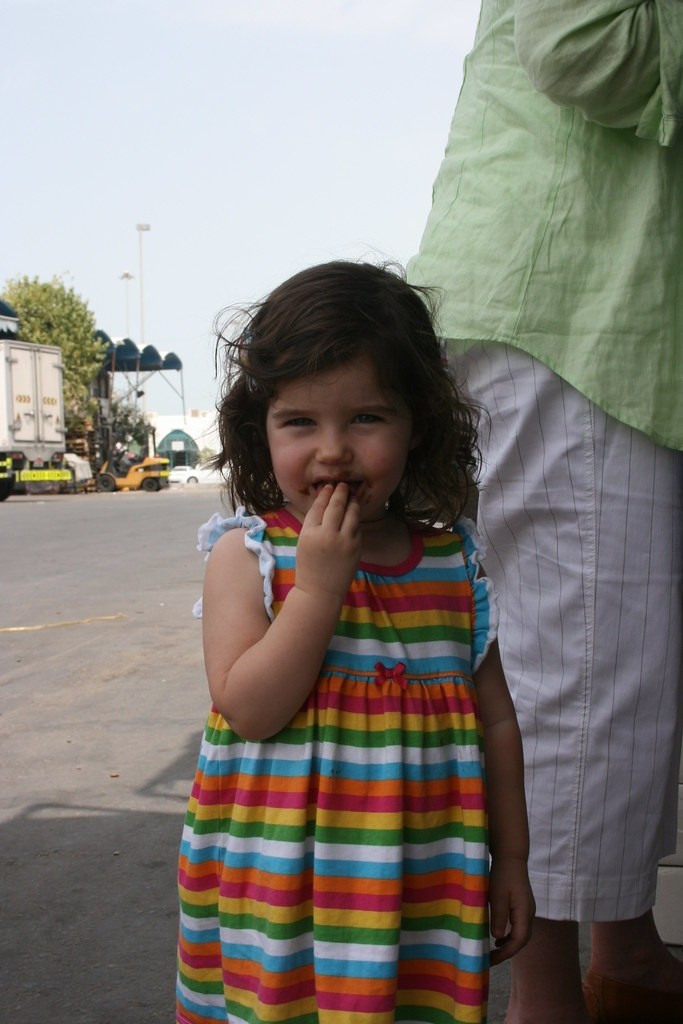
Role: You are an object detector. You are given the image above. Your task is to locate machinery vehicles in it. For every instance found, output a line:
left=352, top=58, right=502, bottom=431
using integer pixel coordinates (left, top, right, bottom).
left=80, top=422, right=170, bottom=492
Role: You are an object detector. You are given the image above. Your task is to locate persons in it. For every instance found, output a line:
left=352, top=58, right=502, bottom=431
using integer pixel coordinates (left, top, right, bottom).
left=172, top=260, right=537, bottom=1024
left=119, top=434, right=140, bottom=468
left=408, top=0, right=683, bottom=1024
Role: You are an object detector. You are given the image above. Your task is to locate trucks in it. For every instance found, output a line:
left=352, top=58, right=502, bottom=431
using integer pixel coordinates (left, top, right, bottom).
left=0, top=339, right=72, bottom=502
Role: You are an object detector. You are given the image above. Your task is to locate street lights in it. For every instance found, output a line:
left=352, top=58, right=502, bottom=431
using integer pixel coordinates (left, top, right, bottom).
left=119, top=272, right=135, bottom=339
left=136, top=223, right=151, bottom=414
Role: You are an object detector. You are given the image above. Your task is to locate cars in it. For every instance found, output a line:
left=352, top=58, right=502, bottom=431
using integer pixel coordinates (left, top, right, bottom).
left=180, top=459, right=234, bottom=485
left=169, top=466, right=192, bottom=481
